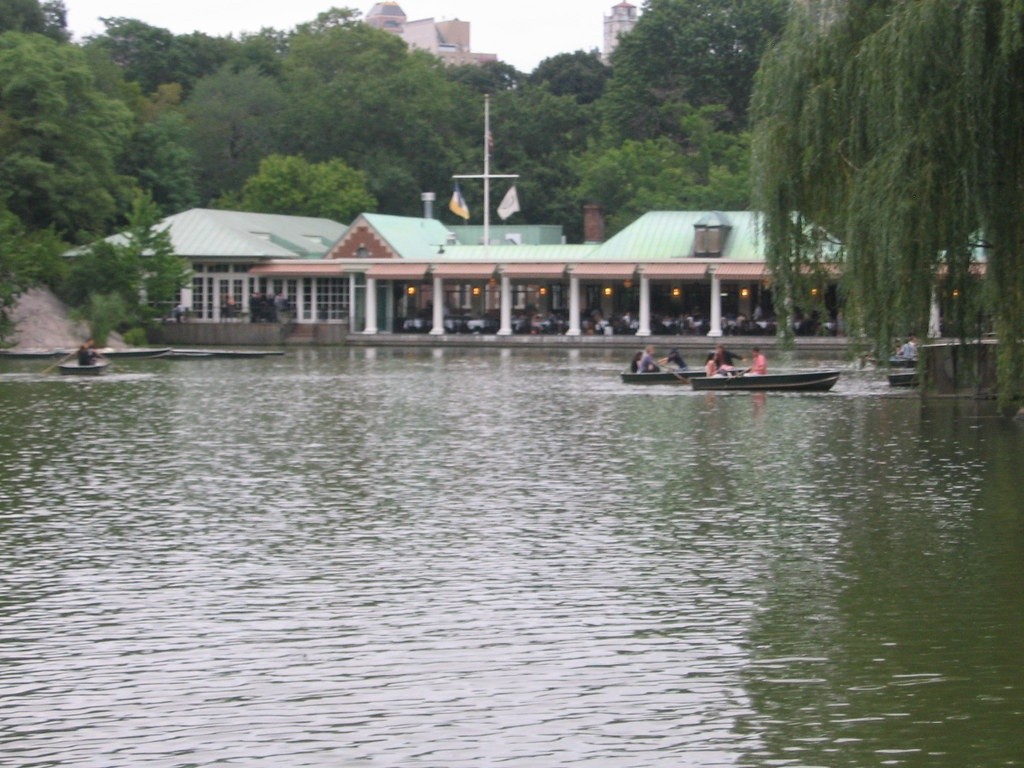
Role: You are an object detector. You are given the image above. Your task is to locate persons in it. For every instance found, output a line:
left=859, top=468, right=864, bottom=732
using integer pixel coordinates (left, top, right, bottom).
left=896, top=333, right=919, bottom=359
left=630, top=344, right=688, bottom=374
left=175, top=293, right=278, bottom=323
left=459, top=304, right=837, bottom=335
left=705, top=343, right=768, bottom=377
left=77, top=339, right=97, bottom=366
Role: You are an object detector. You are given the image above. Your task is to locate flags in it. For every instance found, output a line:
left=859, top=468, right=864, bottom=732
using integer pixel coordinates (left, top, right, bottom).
left=448, top=180, right=471, bottom=220
left=482, top=102, right=493, bottom=156
left=497, top=185, right=521, bottom=221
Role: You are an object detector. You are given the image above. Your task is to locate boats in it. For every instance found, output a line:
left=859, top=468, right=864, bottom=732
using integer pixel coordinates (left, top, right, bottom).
left=57, top=356, right=112, bottom=376
left=619, top=362, right=747, bottom=384
left=686, top=368, right=844, bottom=392
left=886, top=368, right=920, bottom=387
left=859, top=352, right=920, bottom=367
